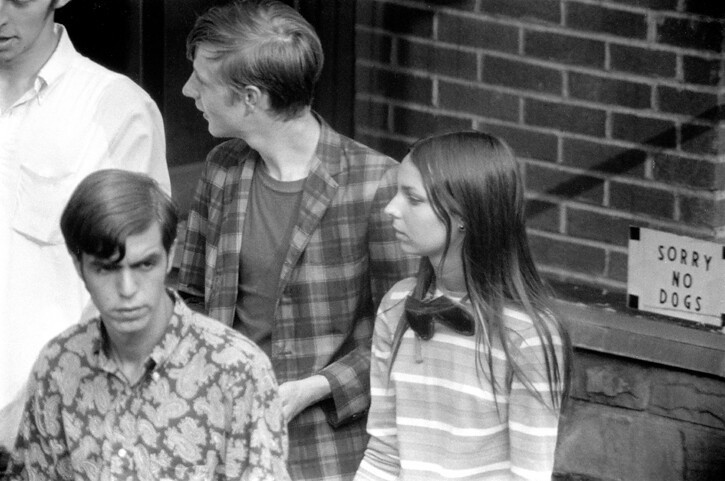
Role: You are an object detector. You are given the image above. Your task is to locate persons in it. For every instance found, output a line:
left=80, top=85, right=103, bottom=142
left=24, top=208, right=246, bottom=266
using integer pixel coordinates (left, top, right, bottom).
left=351, top=131, right=576, bottom=481
left=0, top=169, right=293, bottom=481
left=0, top=0, right=173, bottom=481
left=149, top=0, right=420, bottom=481
